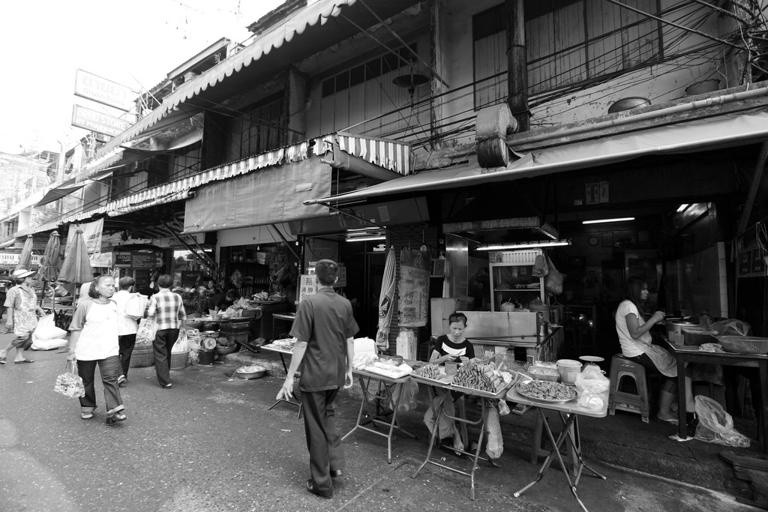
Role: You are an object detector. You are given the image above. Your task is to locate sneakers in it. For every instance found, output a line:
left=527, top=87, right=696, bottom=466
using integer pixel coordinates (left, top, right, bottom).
left=117, top=374, right=126, bottom=387
left=162, top=382, right=173, bottom=388
left=105, top=411, right=128, bottom=425
left=80, top=411, right=94, bottom=419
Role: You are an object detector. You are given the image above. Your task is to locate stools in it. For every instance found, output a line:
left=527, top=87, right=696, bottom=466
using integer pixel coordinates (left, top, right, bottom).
left=607, top=353, right=653, bottom=425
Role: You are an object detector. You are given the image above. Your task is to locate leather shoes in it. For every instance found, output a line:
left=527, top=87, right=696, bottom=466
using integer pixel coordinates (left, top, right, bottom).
left=330, top=468, right=344, bottom=478
left=306, top=478, right=333, bottom=499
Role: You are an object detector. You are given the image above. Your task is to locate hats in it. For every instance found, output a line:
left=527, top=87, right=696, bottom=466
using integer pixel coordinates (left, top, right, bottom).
left=12, top=269, right=37, bottom=280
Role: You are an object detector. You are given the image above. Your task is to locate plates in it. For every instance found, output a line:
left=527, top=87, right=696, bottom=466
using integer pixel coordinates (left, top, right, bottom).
left=513, top=380, right=582, bottom=404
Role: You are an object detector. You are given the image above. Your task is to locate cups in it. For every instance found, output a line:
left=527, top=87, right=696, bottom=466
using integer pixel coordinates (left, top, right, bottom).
left=536, top=320, right=548, bottom=337
left=444, top=360, right=456, bottom=376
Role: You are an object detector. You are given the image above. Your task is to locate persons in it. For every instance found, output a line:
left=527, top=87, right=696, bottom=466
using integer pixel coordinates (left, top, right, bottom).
left=276, top=258, right=360, bottom=499
left=0, top=268, right=44, bottom=367
left=63, top=273, right=188, bottom=427
left=423, top=313, right=486, bottom=454
left=614, top=275, right=679, bottom=423
left=209, top=289, right=234, bottom=313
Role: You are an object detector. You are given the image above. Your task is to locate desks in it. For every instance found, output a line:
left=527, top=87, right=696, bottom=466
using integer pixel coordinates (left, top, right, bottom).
left=413, top=364, right=515, bottom=500
left=509, top=369, right=609, bottom=512
left=645, top=313, right=767, bottom=457
left=341, top=354, right=422, bottom=464
left=264, top=340, right=309, bottom=420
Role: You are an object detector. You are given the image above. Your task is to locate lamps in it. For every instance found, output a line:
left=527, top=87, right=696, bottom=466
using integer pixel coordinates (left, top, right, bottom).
left=583, top=215, right=635, bottom=226
left=472, top=237, right=573, bottom=253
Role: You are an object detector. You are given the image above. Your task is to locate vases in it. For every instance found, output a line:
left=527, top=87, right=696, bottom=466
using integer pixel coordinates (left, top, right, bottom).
left=684, top=77, right=721, bottom=95
left=607, top=96, right=651, bottom=114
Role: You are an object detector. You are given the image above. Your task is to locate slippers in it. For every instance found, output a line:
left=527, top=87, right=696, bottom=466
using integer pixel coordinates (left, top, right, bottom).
left=0, top=356, right=7, bottom=364
left=655, top=415, right=680, bottom=428
left=14, top=358, right=35, bottom=364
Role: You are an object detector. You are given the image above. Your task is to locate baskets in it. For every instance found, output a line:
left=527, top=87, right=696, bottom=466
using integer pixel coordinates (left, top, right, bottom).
left=215, top=340, right=238, bottom=356
left=129, top=343, right=155, bottom=369
left=169, top=350, right=190, bottom=371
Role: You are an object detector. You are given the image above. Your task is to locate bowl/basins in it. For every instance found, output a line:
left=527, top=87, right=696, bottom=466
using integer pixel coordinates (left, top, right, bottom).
left=237, top=369, right=266, bottom=380
left=387, top=355, right=403, bottom=365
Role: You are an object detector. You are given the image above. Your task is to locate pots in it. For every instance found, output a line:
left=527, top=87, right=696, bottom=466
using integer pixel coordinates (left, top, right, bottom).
left=500, top=299, right=564, bottom=325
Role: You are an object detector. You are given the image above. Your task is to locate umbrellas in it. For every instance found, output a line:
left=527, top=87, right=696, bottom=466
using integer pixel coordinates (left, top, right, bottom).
left=14, top=229, right=96, bottom=326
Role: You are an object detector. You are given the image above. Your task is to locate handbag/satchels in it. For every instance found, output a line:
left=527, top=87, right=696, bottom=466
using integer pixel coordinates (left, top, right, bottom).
left=135, top=309, right=160, bottom=344
left=544, top=255, right=565, bottom=296
left=123, top=291, right=149, bottom=322
left=52, top=358, right=86, bottom=399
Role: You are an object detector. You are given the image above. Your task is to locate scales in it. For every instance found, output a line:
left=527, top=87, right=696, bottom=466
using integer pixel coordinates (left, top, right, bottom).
left=579, top=356, right=605, bottom=372
left=199, top=330, right=216, bottom=353
